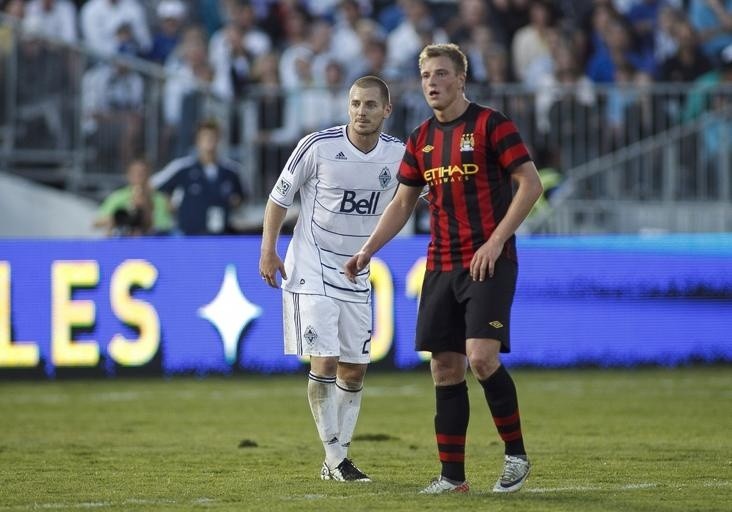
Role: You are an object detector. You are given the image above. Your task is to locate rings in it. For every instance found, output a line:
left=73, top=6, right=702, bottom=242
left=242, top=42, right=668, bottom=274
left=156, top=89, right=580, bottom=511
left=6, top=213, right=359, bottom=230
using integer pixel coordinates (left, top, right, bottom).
left=260, top=276, right=268, bottom=282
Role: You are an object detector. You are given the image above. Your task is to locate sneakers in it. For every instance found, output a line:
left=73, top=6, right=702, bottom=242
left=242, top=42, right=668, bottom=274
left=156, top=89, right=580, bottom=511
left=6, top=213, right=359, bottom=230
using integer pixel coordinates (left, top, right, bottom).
left=492, top=451, right=533, bottom=493
left=319, top=457, right=374, bottom=484
left=416, top=476, right=472, bottom=497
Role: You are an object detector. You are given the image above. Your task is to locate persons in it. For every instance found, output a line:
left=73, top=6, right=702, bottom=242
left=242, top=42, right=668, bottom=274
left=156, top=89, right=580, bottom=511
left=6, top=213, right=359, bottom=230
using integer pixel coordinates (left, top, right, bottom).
left=343, top=41, right=546, bottom=494
left=256, top=74, right=430, bottom=486
